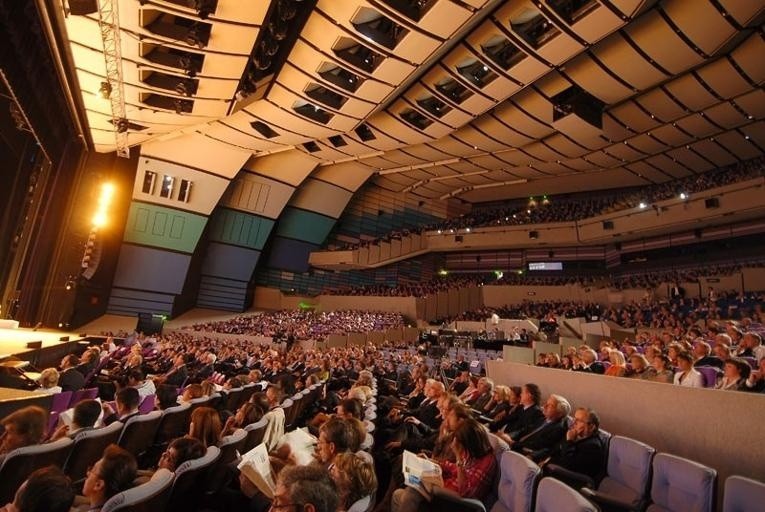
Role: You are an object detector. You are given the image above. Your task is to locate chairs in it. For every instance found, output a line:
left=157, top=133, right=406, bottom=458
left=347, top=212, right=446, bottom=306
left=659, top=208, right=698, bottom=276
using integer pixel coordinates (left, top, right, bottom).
left=0, top=340, right=765, bottom=512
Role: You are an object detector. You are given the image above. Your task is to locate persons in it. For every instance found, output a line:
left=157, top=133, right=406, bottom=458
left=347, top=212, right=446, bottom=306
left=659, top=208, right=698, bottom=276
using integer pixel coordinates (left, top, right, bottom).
left=1, top=155, right=765, bottom=512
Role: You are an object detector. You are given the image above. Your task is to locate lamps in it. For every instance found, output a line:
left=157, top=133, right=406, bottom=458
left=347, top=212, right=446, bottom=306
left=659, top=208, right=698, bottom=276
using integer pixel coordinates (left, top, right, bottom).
left=168, top=1, right=216, bottom=117
left=290, top=2, right=425, bottom=126
left=235, top=1, right=297, bottom=103
left=398, top=1, right=581, bottom=130
